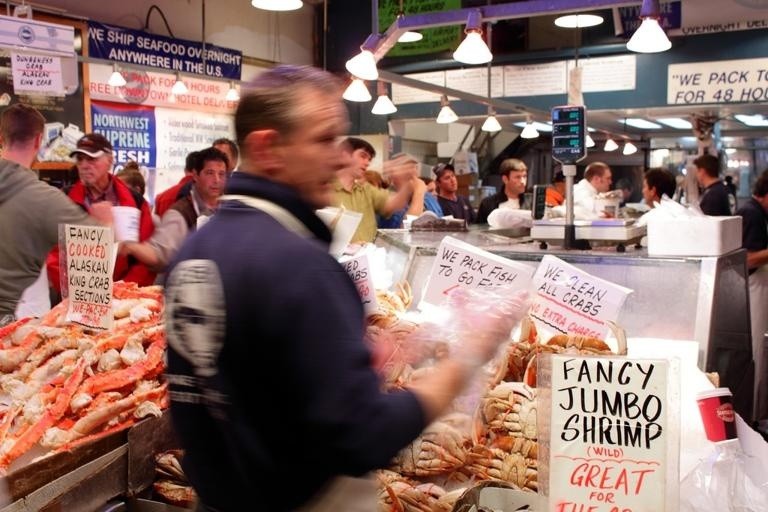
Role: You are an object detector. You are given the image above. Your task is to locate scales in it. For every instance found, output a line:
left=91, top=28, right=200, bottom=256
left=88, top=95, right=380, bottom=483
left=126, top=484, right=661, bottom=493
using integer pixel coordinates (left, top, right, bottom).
left=530, top=104, right=647, bottom=252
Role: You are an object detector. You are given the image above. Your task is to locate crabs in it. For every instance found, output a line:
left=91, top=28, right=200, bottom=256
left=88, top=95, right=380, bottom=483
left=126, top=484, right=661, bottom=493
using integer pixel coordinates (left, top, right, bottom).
left=0, top=275, right=169, bottom=472
left=359, top=280, right=629, bottom=512
left=152, top=448, right=199, bottom=504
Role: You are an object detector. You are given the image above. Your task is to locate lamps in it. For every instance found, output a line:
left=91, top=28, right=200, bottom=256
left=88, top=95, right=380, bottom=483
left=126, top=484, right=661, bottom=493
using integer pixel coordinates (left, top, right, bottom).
left=342, top=1, right=671, bottom=155
left=78, top=58, right=241, bottom=106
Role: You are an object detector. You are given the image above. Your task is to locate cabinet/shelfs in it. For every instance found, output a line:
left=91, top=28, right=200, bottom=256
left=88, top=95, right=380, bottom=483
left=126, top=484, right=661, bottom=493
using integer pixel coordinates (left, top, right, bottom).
left=0, top=8, right=91, bottom=190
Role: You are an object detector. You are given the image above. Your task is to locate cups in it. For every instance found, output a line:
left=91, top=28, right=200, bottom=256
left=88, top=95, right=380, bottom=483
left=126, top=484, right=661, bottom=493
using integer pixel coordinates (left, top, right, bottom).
left=111, top=206, right=142, bottom=243
left=694, top=387, right=741, bottom=446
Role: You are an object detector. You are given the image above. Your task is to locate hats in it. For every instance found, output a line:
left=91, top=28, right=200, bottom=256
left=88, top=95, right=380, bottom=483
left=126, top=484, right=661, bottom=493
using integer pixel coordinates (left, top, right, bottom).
left=431, top=163, right=454, bottom=182
left=69, top=134, right=112, bottom=158
